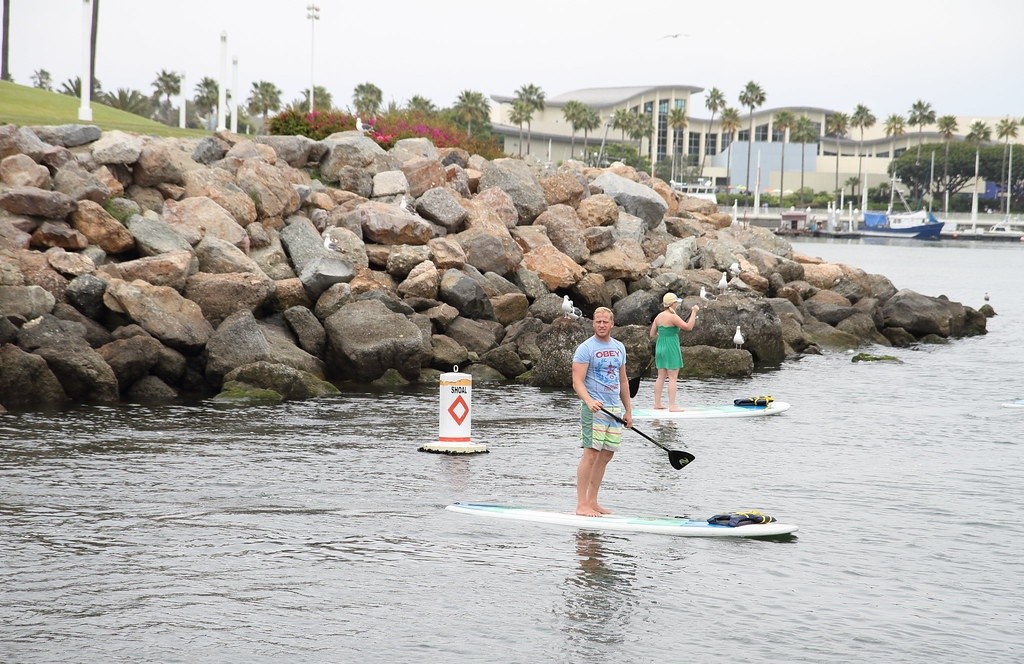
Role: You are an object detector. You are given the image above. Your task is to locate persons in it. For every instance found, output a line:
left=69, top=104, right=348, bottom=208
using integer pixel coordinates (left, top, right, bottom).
left=650, top=293, right=700, bottom=412
left=572, top=307, right=632, bottom=517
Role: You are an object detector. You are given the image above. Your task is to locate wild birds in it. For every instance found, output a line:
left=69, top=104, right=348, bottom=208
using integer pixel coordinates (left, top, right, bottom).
left=984, top=292, right=989, bottom=301
left=356, top=118, right=374, bottom=135
left=700, top=287, right=718, bottom=306
left=324, top=233, right=348, bottom=253
left=562, top=295, right=576, bottom=320
left=400, top=197, right=417, bottom=217
left=718, top=272, right=728, bottom=295
left=733, top=326, right=745, bottom=350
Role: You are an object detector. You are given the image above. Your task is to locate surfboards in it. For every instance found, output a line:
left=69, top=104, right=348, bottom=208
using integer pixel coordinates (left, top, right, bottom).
left=443, top=500, right=802, bottom=541
left=620, top=401, right=791, bottom=421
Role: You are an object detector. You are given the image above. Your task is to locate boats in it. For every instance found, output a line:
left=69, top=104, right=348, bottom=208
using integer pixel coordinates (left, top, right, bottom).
left=816, top=225, right=863, bottom=239
left=856, top=210, right=945, bottom=242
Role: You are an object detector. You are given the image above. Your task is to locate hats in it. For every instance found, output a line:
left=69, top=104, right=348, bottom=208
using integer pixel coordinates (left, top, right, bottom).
left=664, top=292, right=682, bottom=309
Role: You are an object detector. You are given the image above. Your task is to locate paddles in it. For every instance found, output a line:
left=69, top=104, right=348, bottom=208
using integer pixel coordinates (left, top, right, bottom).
left=627, top=310, right=692, bottom=399
left=600, top=406, right=696, bottom=471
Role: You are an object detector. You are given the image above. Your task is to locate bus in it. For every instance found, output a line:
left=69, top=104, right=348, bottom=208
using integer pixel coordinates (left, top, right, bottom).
left=678, top=186, right=718, bottom=204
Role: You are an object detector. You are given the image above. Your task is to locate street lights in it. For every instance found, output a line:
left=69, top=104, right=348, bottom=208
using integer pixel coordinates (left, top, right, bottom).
left=179, top=74, right=188, bottom=129
left=213, top=26, right=241, bottom=136
left=304, top=3, right=320, bottom=112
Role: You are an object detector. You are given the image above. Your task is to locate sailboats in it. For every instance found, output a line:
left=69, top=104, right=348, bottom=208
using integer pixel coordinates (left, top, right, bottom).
left=983, top=140, right=1024, bottom=240
left=939, top=150, right=987, bottom=240
